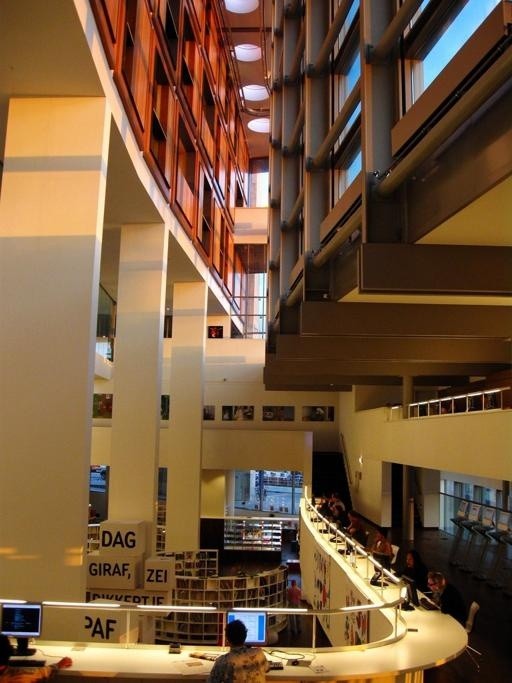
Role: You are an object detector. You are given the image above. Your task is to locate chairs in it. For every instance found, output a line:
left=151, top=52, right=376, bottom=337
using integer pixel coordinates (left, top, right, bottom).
left=462, top=601, right=485, bottom=674
left=390, top=544, right=401, bottom=570
left=447, top=500, right=512, bottom=588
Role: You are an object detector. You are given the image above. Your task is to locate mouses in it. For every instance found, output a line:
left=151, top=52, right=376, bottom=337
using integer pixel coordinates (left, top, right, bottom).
left=292, top=661, right=299, bottom=666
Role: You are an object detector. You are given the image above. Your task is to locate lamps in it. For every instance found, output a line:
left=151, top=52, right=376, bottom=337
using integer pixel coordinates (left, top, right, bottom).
left=358, top=456, right=363, bottom=464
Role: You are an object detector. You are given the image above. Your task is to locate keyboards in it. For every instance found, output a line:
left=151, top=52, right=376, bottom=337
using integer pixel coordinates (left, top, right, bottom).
left=418, top=598, right=440, bottom=610
left=385, top=578, right=396, bottom=584
left=9, top=660, right=46, bottom=667
left=268, top=661, right=283, bottom=670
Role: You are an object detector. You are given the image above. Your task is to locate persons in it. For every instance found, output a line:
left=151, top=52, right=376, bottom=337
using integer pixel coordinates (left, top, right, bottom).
left=311, top=492, right=368, bottom=555
left=394, top=549, right=433, bottom=601
left=0, top=634, right=73, bottom=683
left=427, top=571, right=468, bottom=628
left=287, top=580, right=302, bottom=633
left=362, top=527, right=395, bottom=566
left=283, top=521, right=297, bottom=529
left=88, top=504, right=96, bottom=524
left=207, top=620, right=271, bottom=683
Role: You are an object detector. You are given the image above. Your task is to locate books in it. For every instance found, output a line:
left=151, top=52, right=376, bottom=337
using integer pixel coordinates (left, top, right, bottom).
left=155, top=550, right=288, bottom=640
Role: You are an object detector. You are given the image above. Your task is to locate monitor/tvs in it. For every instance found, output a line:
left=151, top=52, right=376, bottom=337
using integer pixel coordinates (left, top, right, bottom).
left=397, top=575, right=420, bottom=611
left=370, top=551, right=391, bottom=586
left=307, top=495, right=362, bottom=555
left=226, top=610, right=269, bottom=648
left=0, top=603, right=43, bottom=656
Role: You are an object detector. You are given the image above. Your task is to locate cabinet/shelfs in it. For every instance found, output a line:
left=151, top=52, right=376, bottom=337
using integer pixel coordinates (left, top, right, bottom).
left=85, top=496, right=290, bottom=645
